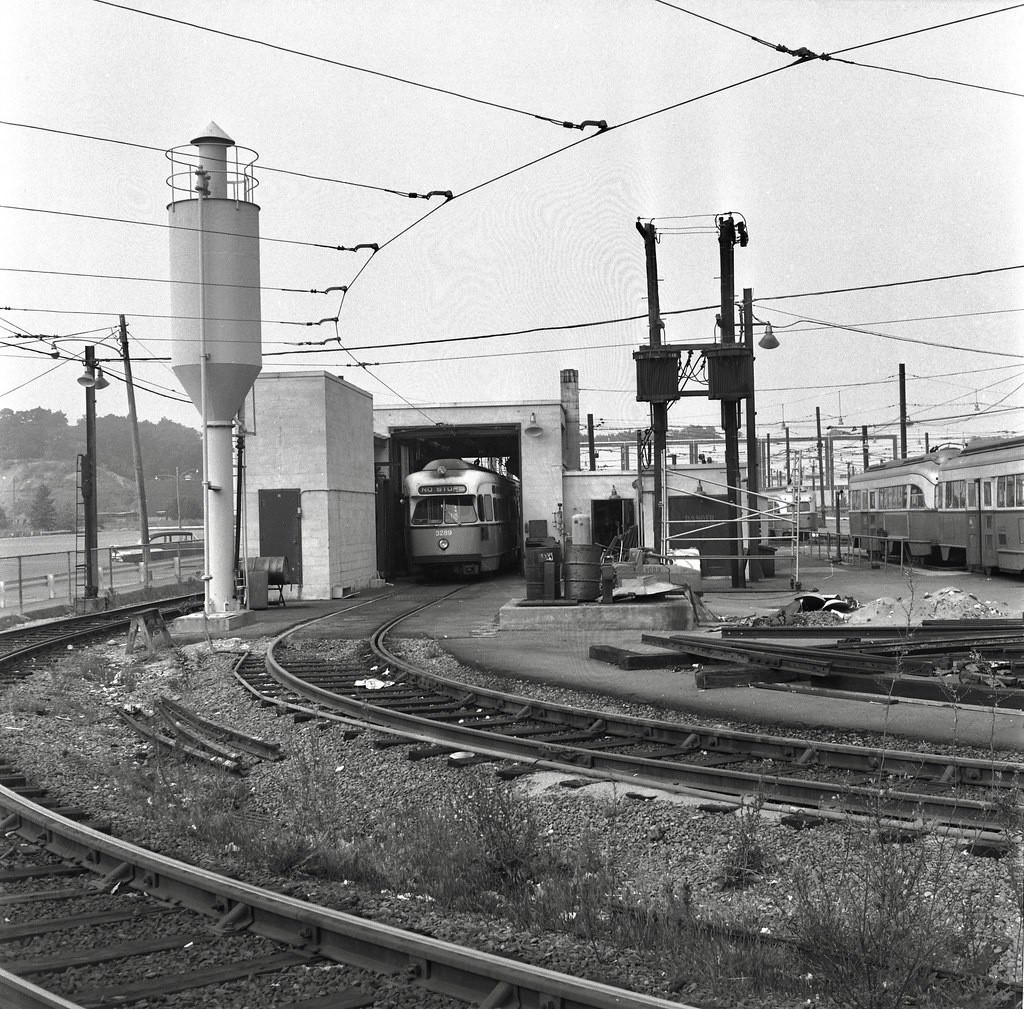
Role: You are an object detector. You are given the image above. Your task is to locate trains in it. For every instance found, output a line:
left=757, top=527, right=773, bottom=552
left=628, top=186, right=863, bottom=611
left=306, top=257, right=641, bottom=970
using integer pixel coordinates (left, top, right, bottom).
left=850, top=434, right=1023, bottom=580
left=767, top=485, right=815, bottom=538
left=401, top=458, right=521, bottom=579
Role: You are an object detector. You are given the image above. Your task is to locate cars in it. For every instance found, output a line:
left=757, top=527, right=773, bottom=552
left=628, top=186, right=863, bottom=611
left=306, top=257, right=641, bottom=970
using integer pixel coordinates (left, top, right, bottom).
left=110, top=529, right=204, bottom=564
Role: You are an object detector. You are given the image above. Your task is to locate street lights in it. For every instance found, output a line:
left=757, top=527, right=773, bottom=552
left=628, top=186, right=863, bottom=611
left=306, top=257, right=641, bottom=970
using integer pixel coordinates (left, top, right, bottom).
left=75, top=347, right=110, bottom=598
left=717, top=310, right=781, bottom=589
left=155, top=465, right=200, bottom=527
